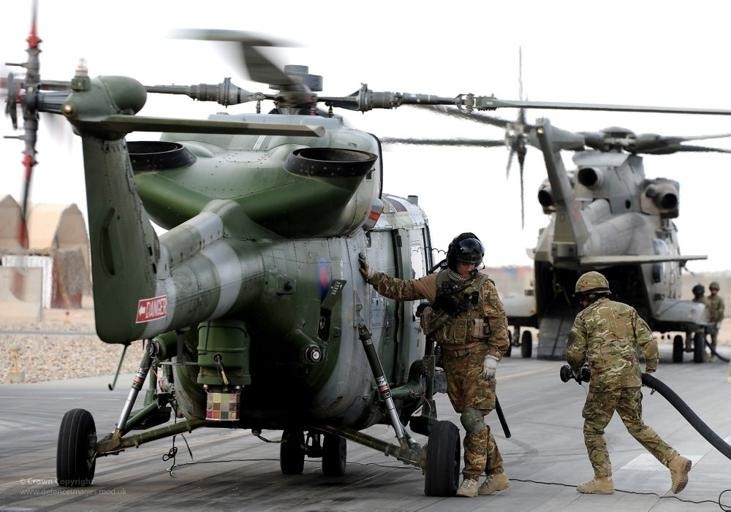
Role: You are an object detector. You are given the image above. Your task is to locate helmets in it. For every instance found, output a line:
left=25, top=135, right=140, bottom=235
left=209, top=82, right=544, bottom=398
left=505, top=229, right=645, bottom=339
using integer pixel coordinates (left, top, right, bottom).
left=575, top=271, right=611, bottom=295
left=447, top=232, right=485, bottom=271
left=709, top=281, right=720, bottom=291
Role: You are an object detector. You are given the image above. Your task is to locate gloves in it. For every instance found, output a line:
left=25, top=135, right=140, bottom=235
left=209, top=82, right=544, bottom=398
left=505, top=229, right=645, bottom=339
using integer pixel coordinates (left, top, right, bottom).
left=479, top=354, right=499, bottom=380
left=357, top=252, right=376, bottom=280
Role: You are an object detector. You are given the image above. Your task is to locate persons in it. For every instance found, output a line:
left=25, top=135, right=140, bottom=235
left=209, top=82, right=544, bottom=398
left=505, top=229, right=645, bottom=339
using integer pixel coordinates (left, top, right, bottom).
left=357, top=231, right=512, bottom=499
left=565, top=268, right=694, bottom=495
left=706, top=282, right=726, bottom=360
left=690, top=284, right=714, bottom=323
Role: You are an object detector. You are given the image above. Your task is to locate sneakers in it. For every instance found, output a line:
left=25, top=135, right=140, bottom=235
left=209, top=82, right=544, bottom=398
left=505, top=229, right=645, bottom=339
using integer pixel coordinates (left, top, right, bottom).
left=456, top=471, right=486, bottom=498
left=668, top=453, right=692, bottom=494
left=575, top=476, right=614, bottom=495
left=479, top=471, right=510, bottom=496
